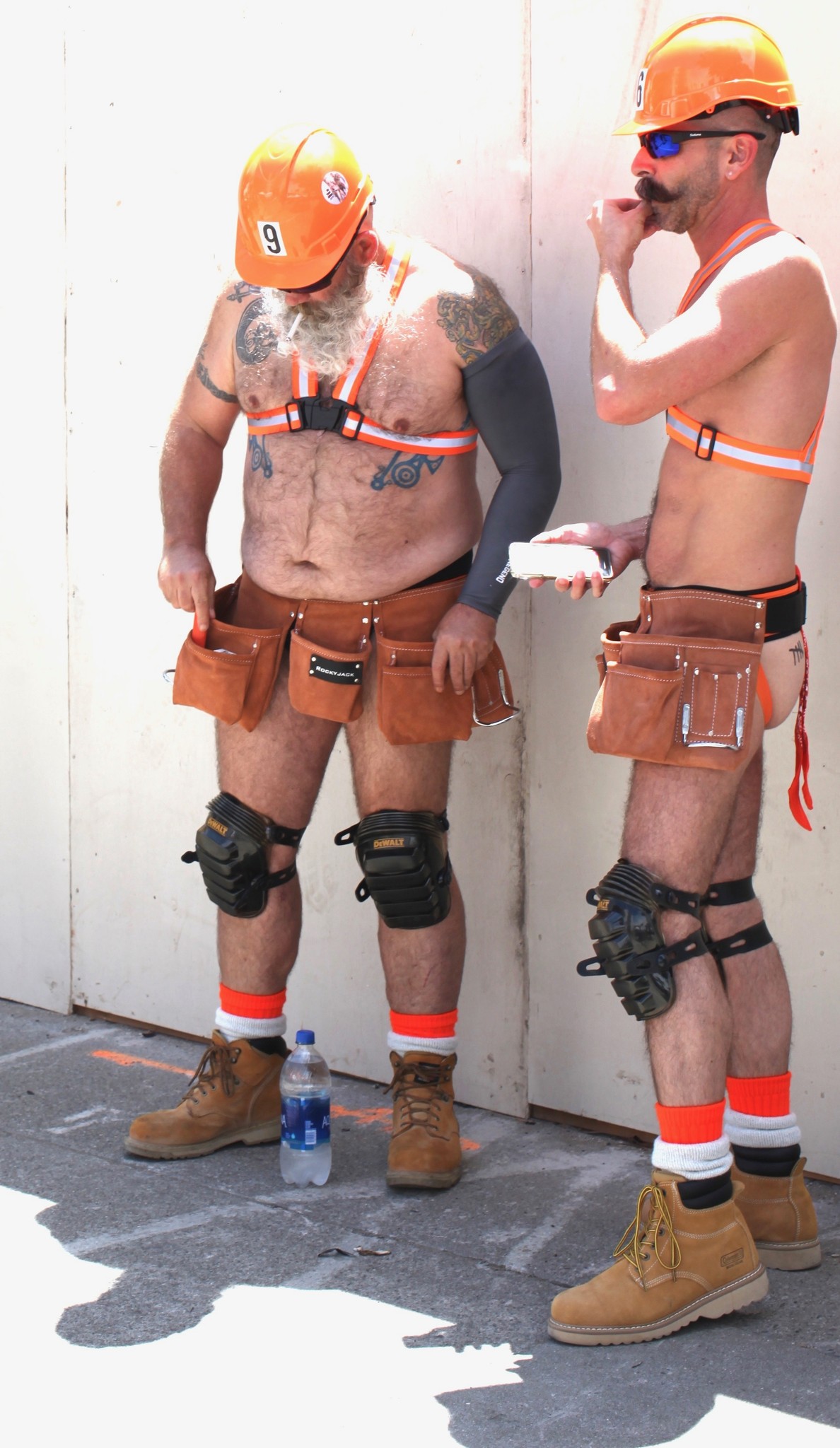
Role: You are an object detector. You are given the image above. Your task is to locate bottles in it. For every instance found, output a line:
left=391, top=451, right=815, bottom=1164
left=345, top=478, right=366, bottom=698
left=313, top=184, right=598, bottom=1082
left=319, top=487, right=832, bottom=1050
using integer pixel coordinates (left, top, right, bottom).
left=278, top=1030, right=333, bottom=1187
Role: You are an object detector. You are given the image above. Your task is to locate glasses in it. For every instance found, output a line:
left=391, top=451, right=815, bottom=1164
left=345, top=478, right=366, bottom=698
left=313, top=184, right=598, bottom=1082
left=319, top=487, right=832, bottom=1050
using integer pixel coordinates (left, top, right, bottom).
left=639, top=130, right=766, bottom=159
left=278, top=210, right=368, bottom=294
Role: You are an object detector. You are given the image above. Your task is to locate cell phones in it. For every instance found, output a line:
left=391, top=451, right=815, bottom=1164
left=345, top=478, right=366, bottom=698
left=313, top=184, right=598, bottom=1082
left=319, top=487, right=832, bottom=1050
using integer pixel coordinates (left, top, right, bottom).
left=508, top=541, right=614, bottom=582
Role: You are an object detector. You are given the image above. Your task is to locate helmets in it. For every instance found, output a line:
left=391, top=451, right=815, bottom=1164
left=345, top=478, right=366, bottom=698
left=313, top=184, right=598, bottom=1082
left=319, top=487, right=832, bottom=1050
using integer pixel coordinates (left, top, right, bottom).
left=235, top=122, right=376, bottom=289
left=611, top=11, right=802, bottom=136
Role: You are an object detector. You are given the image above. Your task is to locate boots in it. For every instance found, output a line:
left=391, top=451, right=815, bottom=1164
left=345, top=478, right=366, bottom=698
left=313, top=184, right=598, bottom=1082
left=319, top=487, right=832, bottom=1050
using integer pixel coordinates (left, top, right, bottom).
left=382, top=1051, right=463, bottom=1189
left=729, top=1145, right=822, bottom=1271
left=124, top=1029, right=293, bottom=1160
left=548, top=1167, right=769, bottom=1346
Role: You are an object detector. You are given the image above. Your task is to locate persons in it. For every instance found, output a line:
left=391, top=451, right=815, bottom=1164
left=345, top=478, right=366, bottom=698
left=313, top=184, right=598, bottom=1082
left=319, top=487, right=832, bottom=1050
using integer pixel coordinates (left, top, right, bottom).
left=514, top=14, right=838, bottom=1346
left=121, top=133, right=569, bottom=1194
